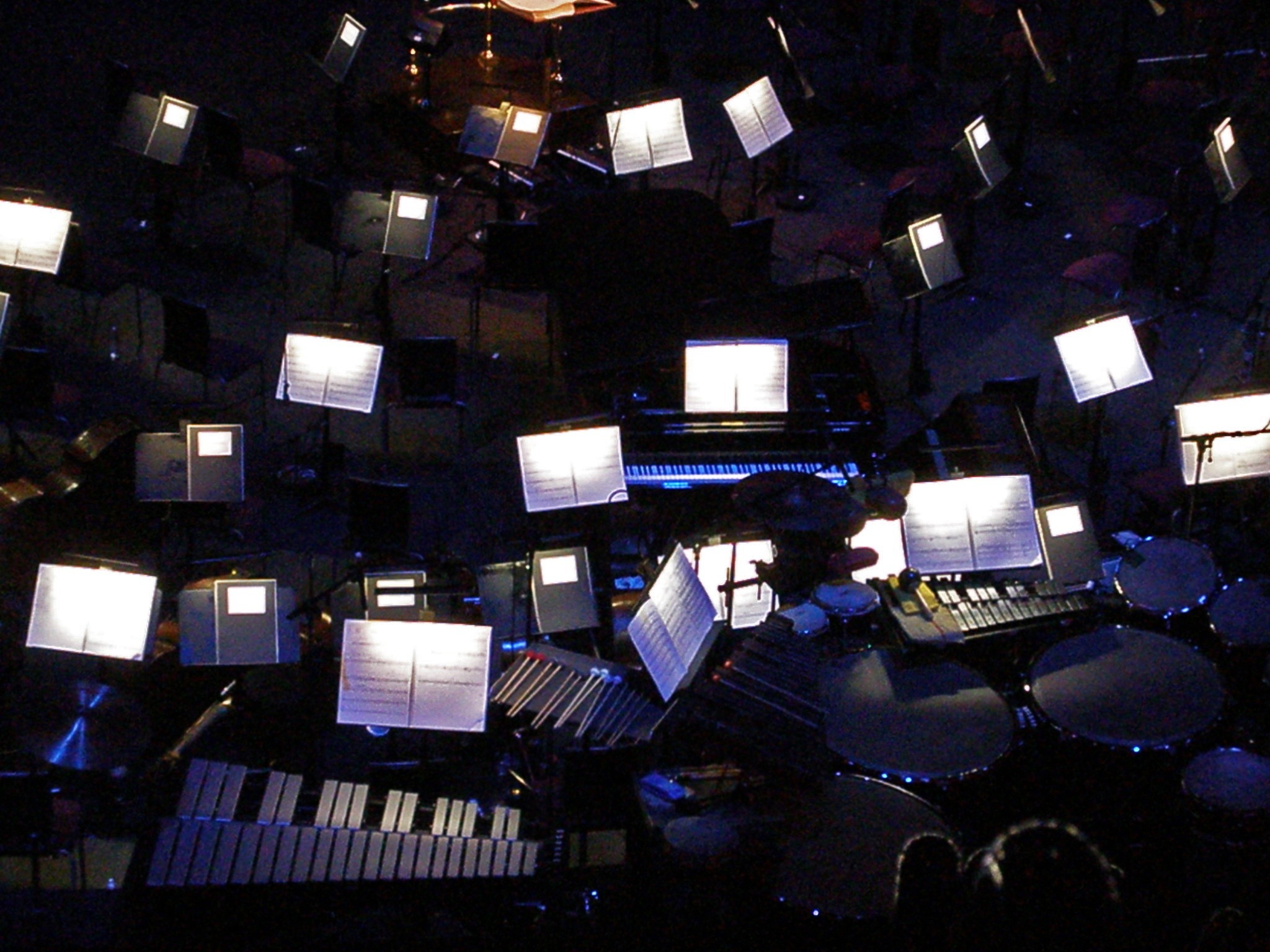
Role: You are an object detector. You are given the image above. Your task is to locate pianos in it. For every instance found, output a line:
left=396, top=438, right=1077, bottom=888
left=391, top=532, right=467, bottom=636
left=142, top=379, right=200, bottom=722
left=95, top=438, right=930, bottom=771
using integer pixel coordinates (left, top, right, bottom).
left=868, top=565, right=1094, bottom=651
left=537, top=184, right=892, bottom=488
left=120, top=752, right=541, bottom=891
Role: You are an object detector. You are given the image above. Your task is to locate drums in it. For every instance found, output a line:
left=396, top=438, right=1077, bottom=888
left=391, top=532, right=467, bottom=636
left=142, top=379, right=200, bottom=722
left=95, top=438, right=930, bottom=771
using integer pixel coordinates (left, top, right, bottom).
left=1025, top=624, right=1230, bottom=756
left=1207, top=573, right=1269, bottom=648
left=1111, top=531, right=1227, bottom=618
left=770, top=770, right=965, bottom=924
left=817, top=642, right=1021, bottom=787
left=1182, top=740, right=1269, bottom=853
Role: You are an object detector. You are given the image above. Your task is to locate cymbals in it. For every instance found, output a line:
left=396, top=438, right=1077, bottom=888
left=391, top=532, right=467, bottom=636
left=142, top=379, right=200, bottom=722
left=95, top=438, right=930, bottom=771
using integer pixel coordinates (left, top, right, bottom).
left=18, top=675, right=152, bottom=771
